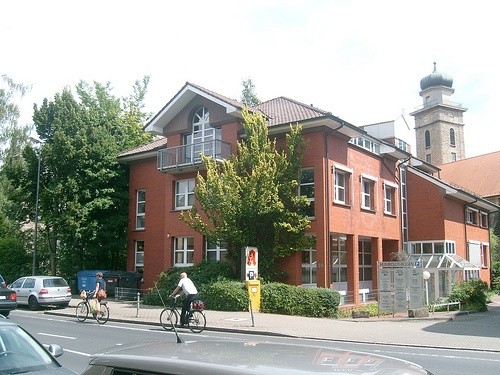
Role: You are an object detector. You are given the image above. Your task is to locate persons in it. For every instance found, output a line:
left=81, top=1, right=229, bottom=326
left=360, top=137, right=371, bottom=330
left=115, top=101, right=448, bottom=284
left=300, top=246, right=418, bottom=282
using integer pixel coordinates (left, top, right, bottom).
left=170, top=272, right=198, bottom=326
left=86, top=272, right=107, bottom=318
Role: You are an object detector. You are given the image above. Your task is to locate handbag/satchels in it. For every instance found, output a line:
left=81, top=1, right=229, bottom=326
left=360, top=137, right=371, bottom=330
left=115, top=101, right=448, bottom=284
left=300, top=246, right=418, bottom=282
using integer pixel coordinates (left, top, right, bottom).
left=97, top=290, right=106, bottom=298
left=193, top=300, right=204, bottom=309
left=91, top=298, right=100, bottom=314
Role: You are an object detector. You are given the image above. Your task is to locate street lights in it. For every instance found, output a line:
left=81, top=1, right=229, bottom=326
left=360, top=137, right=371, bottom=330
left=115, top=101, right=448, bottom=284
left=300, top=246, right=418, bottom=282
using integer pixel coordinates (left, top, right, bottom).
left=28, top=134, right=43, bottom=277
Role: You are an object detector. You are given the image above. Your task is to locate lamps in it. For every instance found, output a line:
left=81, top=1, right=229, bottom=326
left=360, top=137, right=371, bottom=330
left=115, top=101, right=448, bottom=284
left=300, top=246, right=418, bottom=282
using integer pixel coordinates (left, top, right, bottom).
left=167, top=232, right=171, bottom=239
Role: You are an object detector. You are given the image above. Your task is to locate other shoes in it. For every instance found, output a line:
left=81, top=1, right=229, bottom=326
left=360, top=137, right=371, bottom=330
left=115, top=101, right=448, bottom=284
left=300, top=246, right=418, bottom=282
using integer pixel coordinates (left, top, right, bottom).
left=189, top=314, right=193, bottom=317
left=178, top=325, right=184, bottom=328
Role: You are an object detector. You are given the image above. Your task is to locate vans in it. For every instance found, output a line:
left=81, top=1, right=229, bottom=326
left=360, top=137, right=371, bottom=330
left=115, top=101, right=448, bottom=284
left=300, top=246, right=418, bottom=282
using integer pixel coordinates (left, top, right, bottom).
left=80, top=334, right=435, bottom=375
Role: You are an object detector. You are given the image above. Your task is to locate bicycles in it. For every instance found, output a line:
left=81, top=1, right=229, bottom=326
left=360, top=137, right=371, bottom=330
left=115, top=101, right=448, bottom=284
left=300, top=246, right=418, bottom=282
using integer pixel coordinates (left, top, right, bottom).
left=160, top=294, right=206, bottom=334
left=76, top=289, right=109, bottom=324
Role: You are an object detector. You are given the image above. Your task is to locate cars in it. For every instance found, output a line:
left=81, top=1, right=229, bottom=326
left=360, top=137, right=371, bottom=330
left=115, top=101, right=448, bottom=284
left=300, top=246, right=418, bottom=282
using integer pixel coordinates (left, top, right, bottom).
left=0, top=274, right=18, bottom=316
left=0, top=313, right=80, bottom=375
left=7, top=275, right=73, bottom=311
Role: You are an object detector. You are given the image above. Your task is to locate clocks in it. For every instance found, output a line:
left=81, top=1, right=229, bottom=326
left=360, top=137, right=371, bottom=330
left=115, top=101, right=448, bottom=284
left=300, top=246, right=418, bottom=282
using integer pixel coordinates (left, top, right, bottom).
left=423, top=113, right=434, bottom=122
left=448, top=112, right=455, bottom=121
